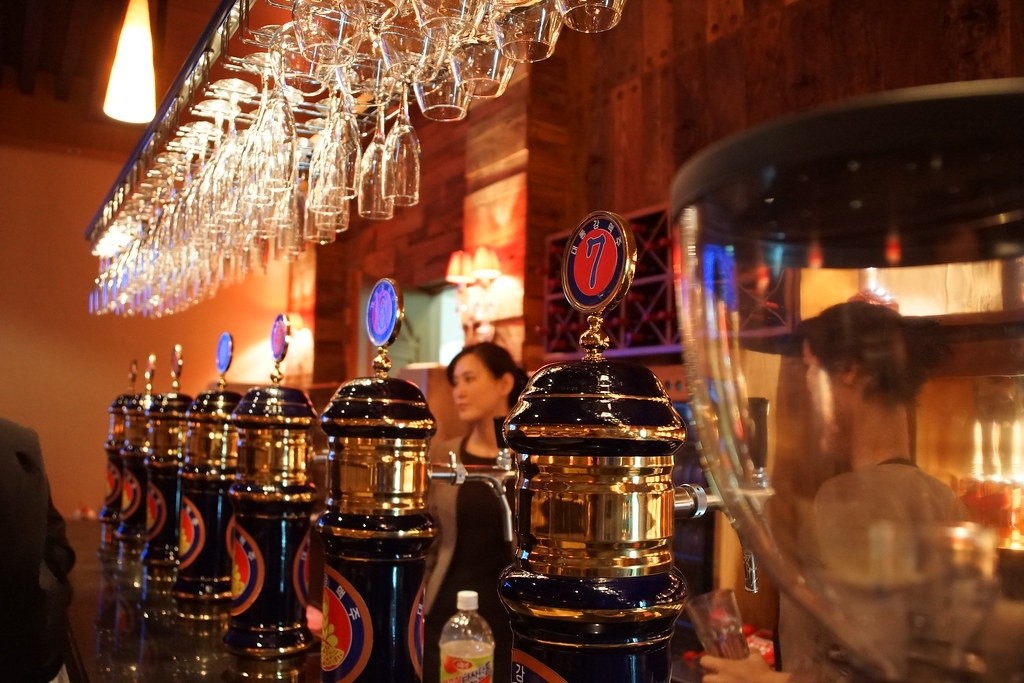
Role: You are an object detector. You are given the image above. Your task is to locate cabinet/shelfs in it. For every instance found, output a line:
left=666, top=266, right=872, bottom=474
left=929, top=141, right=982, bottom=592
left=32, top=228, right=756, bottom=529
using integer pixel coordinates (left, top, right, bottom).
left=545, top=204, right=794, bottom=364
left=919, top=309, right=1024, bottom=603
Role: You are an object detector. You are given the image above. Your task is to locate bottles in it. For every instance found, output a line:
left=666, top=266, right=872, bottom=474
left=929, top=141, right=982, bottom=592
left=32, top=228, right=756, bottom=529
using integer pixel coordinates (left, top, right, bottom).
left=439, top=590, right=495, bottom=683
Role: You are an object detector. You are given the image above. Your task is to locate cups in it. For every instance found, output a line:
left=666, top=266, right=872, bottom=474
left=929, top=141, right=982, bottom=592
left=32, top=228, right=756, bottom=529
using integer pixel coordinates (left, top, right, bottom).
left=292, top=0, right=626, bottom=99
left=685, top=590, right=750, bottom=660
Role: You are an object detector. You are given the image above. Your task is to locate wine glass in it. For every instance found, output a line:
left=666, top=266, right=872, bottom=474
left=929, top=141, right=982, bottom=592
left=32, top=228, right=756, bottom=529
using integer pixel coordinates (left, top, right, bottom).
left=87, top=0, right=420, bottom=319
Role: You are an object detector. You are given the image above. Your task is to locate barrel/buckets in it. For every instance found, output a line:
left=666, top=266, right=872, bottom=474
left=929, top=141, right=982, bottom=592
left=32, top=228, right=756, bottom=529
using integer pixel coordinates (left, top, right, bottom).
left=98, top=332, right=240, bottom=599
left=322, top=278, right=440, bottom=683
left=496, top=217, right=685, bottom=683
left=224, top=314, right=318, bottom=659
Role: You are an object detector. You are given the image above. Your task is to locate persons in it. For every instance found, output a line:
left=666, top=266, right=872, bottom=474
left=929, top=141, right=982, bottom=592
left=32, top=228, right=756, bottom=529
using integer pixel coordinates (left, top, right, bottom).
left=421, top=343, right=530, bottom=683
left=700, top=302, right=969, bottom=683
left=0, top=419, right=76, bottom=683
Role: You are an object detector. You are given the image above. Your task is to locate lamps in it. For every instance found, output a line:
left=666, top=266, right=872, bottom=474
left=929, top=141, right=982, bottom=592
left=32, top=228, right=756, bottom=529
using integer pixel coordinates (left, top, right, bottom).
left=101, top=0, right=157, bottom=124
left=445, top=247, right=503, bottom=344
